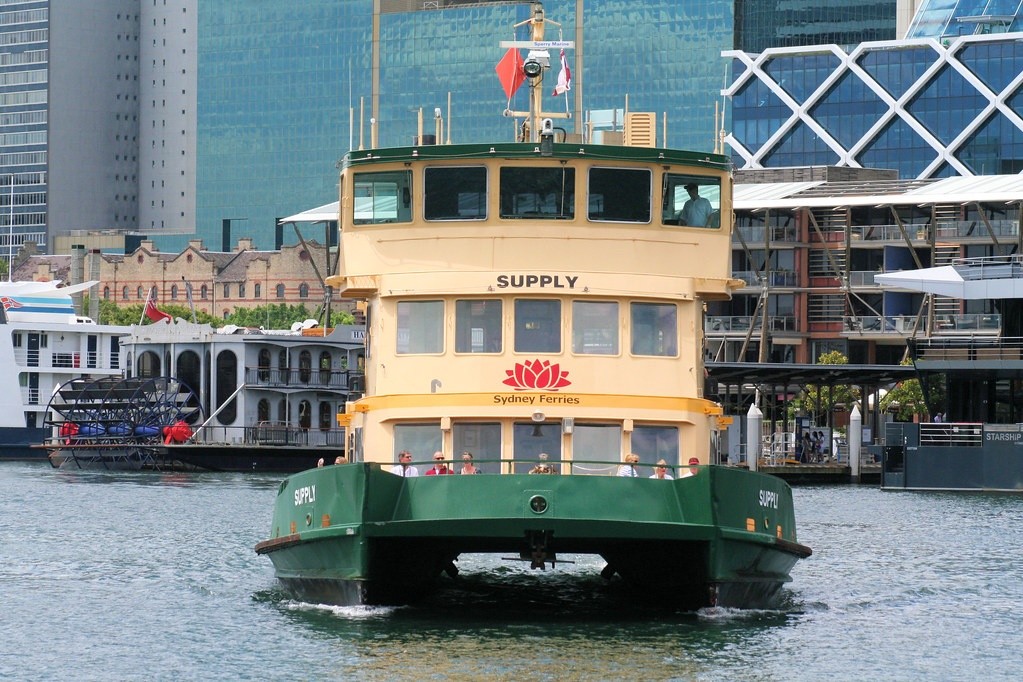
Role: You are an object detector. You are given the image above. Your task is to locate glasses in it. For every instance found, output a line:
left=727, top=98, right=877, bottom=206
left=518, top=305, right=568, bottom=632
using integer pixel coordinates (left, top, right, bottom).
left=435, top=457, right=444, bottom=460
left=631, top=460, right=638, bottom=462
left=403, top=455, right=411, bottom=458
left=687, top=188, right=693, bottom=192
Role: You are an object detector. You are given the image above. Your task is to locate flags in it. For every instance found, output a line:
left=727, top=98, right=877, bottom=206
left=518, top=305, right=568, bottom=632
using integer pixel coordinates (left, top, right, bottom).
left=146, top=299, right=172, bottom=325
left=552, top=49, right=571, bottom=96
left=1, top=298, right=23, bottom=310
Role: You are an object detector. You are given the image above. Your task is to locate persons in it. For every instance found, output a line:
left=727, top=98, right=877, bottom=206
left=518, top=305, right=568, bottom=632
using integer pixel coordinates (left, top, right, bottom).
left=391, top=450, right=419, bottom=476
left=650, top=459, right=673, bottom=480
left=803, top=430, right=826, bottom=465
left=679, top=183, right=714, bottom=228
left=318, top=457, right=348, bottom=467
left=619, top=454, right=640, bottom=478
left=934, top=413, right=941, bottom=423
left=685, top=458, right=699, bottom=478
left=458, top=452, right=482, bottom=474
left=426, top=451, right=454, bottom=474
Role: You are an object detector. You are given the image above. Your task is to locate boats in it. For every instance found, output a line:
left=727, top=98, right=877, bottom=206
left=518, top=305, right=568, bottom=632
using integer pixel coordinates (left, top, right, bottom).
left=250, top=0, right=815, bottom=620
left=0, top=277, right=132, bottom=460
left=26, top=316, right=417, bottom=473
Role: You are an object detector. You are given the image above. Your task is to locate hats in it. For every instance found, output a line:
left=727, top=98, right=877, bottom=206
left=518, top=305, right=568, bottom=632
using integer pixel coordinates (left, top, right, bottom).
left=689, top=458, right=699, bottom=465
left=684, top=182, right=698, bottom=189
